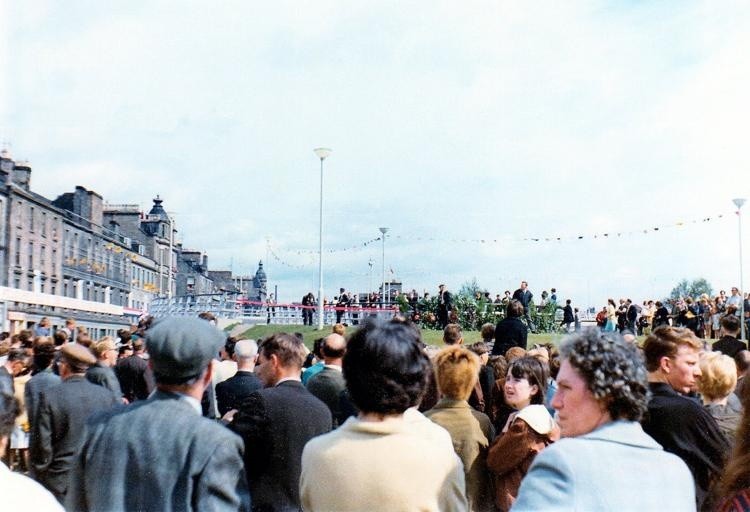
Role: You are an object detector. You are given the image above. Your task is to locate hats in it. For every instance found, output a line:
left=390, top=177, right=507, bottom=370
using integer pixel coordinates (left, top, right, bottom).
left=145, top=318, right=226, bottom=378
left=60, top=343, right=97, bottom=364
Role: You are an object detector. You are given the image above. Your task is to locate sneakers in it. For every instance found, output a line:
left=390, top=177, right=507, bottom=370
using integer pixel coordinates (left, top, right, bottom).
left=531, top=330, right=538, bottom=334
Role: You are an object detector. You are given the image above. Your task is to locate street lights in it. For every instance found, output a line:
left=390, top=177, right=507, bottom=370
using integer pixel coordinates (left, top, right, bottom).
left=166, top=211, right=178, bottom=298
left=312, top=147, right=332, bottom=331
left=378, top=228, right=390, bottom=310
left=159, top=245, right=165, bottom=298
left=732, top=199, right=746, bottom=340
